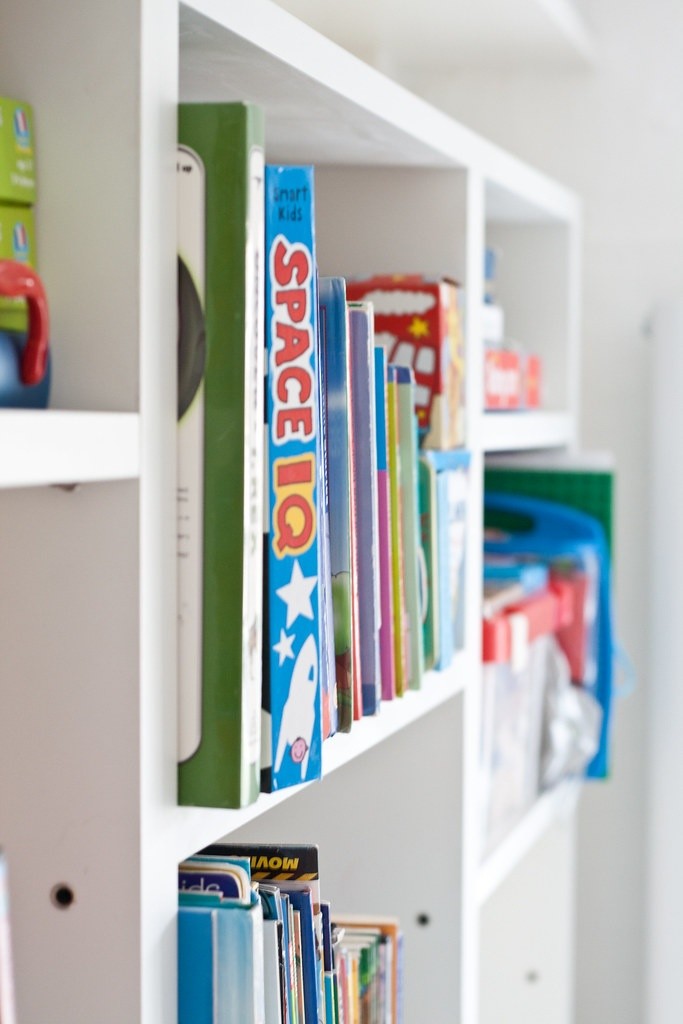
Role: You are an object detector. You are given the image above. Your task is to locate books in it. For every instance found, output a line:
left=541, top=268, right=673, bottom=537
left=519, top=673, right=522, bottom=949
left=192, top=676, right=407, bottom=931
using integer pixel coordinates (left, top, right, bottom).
left=178, top=844, right=407, bottom=1024
left=171, top=96, right=431, bottom=804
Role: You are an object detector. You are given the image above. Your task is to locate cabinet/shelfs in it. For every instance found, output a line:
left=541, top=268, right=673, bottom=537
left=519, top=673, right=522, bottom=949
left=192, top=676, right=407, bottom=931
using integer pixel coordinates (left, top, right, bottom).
left=0, top=0, right=604, bottom=1024
